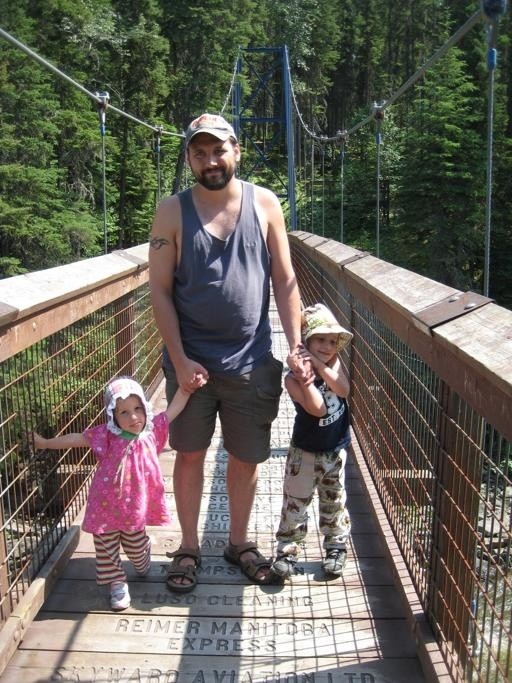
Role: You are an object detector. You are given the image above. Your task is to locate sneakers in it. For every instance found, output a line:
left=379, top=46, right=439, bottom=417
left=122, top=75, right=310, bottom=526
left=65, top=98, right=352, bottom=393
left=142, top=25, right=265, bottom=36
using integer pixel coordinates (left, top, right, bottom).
left=111, top=581, right=132, bottom=610
left=321, top=549, right=348, bottom=578
left=271, top=552, right=299, bottom=579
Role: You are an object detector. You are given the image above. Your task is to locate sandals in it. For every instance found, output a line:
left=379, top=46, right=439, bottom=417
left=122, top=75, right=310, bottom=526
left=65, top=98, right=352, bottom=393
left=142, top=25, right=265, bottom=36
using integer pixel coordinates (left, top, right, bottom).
left=223, top=537, right=284, bottom=586
left=166, top=545, right=202, bottom=593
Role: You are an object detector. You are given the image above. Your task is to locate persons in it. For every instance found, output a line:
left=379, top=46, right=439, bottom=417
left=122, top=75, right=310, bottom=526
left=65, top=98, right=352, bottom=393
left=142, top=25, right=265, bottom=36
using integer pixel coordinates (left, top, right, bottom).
left=24, top=374, right=206, bottom=611
left=269, top=304, right=351, bottom=581
left=148, top=113, right=311, bottom=595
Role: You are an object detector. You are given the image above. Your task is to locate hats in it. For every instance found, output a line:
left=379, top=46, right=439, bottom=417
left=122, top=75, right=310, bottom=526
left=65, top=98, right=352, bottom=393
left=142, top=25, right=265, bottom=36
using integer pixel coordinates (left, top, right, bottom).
left=185, top=113, right=237, bottom=148
left=301, top=303, right=353, bottom=354
left=104, top=378, right=154, bottom=438
